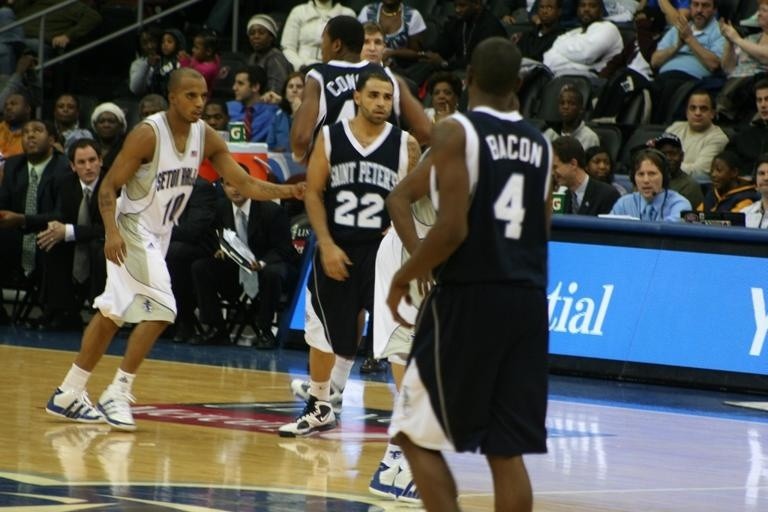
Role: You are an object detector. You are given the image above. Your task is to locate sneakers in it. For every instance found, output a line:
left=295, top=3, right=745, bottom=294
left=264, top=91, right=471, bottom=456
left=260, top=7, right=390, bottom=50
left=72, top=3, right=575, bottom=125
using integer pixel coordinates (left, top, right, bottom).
left=278, top=389, right=335, bottom=438
left=368, top=462, right=423, bottom=502
left=289, top=378, right=344, bottom=416
left=359, top=359, right=387, bottom=372
left=45, top=387, right=105, bottom=423
left=95, top=387, right=136, bottom=431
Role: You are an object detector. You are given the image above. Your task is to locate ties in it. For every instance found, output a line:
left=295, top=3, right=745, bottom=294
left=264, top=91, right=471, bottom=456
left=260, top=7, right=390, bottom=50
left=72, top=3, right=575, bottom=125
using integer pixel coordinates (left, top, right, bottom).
left=571, top=192, right=577, bottom=214
left=72, top=188, right=90, bottom=283
left=238, top=210, right=258, bottom=299
left=643, top=204, right=652, bottom=221
left=242, top=107, right=252, bottom=141
left=21, top=168, right=37, bottom=273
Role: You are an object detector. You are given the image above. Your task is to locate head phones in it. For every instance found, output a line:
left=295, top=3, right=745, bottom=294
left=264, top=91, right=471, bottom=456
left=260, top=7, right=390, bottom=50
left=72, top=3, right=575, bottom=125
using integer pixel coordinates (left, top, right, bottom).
left=752, top=160, right=768, bottom=184
left=629, top=148, right=670, bottom=189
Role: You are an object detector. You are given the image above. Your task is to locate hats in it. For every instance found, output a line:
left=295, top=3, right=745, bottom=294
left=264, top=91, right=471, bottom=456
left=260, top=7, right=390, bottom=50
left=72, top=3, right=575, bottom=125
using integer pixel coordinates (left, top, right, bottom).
left=654, top=133, right=680, bottom=149
left=246, top=13, right=277, bottom=39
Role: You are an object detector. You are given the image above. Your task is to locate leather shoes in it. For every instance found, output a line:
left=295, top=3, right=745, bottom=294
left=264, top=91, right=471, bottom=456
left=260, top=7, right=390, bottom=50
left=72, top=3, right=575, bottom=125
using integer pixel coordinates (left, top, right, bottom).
left=24, top=314, right=275, bottom=349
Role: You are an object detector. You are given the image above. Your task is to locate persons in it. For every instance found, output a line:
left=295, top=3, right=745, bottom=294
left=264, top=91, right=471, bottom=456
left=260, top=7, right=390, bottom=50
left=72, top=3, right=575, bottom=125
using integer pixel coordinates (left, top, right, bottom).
left=149, top=167, right=214, bottom=342
left=276, top=63, right=423, bottom=439
left=387, top=36, right=554, bottom=511
left=367, top=95, right=517, bottom=501
left=290, top=14, right=435, bottom=414
left=307, top=23, right=438, bottom=374
left=1, top=138, right=122, bottom=338
left=2, top=117, right=73, bottom=333
left=44, top=66, right=306, bottom=434
left=191, top=164, right=299, bottom=350
left=0, top=1, right=767, bottom=228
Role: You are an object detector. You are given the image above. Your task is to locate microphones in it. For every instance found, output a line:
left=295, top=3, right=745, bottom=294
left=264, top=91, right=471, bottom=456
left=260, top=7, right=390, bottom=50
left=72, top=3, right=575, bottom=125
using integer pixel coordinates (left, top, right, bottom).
left=652, top=189, right=667, bottom=196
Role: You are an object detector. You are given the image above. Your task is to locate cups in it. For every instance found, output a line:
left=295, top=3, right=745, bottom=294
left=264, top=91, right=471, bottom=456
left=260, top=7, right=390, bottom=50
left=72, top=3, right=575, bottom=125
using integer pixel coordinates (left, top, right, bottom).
left=551, top=193, right=565, bottom=214
left=228, top=122, right=245, bottom=143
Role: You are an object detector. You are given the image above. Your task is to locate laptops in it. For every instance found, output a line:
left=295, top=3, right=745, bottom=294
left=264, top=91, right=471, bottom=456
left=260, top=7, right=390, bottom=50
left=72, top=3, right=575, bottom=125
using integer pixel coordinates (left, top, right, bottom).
left=680, top=210, right=745, bottom=227
